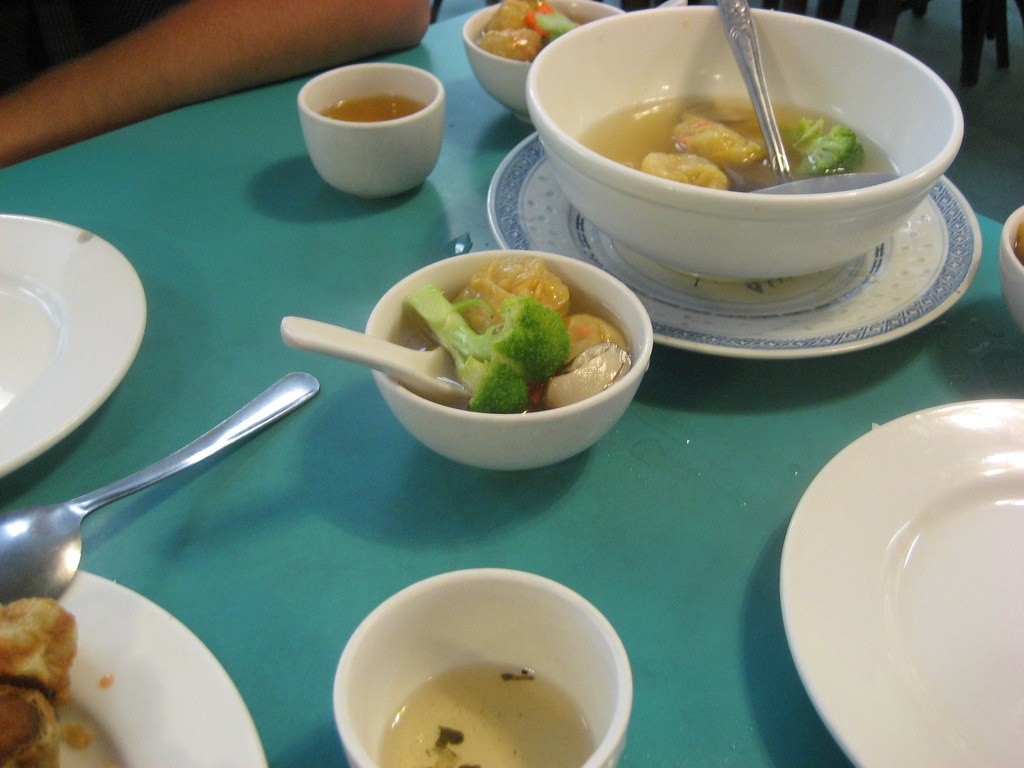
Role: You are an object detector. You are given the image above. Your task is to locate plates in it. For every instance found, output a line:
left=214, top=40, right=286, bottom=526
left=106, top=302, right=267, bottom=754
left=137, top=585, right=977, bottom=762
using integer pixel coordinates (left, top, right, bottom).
left=58, top=570, right=268, bottom=768
left=779, top=397, right=1024, bottom=768
left=0, top=213, right=147, bottom=475
left=486, top=132, right=981, bottom=359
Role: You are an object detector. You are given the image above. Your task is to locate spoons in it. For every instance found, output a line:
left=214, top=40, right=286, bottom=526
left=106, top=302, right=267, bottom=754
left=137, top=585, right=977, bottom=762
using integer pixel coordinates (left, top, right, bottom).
left=0, top=372, right=320, bottom=610
left=280, top=315, right=471, bottom=396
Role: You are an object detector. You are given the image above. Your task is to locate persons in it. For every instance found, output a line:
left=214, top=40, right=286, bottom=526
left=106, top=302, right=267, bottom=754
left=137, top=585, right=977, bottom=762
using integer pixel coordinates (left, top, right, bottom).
left=0, top=0, right=432, bottom=168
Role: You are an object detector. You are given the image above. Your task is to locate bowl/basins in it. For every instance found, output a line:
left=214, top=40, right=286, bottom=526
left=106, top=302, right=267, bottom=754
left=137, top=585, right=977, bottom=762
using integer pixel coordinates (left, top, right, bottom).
left=462, top=0, right=625, bottom=125
left=297, top=61, right=444, bottom=198
left=365, top=249, right=653, bottom=471
left=333, top=567, right=633, bottom=768
left=525, top=6, right=964, bottom=282
left=999, top=205, right=1024, bottom=334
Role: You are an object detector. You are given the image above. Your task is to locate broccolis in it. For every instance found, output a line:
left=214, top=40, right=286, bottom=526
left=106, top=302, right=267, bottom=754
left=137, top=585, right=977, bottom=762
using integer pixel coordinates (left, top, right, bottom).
left=787, top=115, right=866, bottom=179
left=537, top=11, right=577, bottom=40
left=405, top=285, right=571, bottom=415
